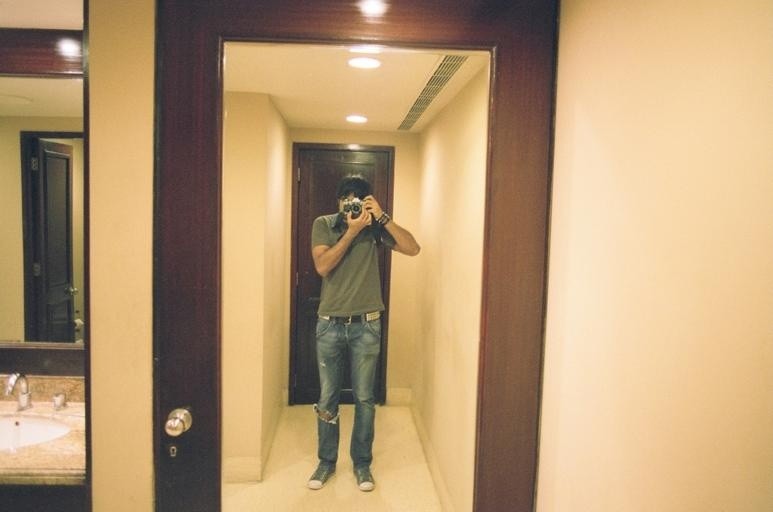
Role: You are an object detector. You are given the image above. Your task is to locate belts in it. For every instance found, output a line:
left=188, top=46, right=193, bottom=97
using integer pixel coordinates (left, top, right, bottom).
left=319, top=311, right=380, bottom=325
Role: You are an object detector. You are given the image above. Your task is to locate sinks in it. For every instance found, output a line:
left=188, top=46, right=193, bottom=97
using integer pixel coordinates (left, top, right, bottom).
left=0, top=412, right=72, bottom=451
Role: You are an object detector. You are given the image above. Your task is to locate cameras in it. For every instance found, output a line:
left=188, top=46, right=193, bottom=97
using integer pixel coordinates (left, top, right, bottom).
left=343, top=197, right=363, bottom=215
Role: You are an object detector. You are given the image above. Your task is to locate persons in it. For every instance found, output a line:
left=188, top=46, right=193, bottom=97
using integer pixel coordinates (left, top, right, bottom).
left=307, top=176, right=420, bottom=492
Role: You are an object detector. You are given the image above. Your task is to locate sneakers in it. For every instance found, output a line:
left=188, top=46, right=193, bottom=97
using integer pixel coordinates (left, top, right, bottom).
left=309, top=461, right=335, bottom=489
left=354, top=463, right=374, bottom=490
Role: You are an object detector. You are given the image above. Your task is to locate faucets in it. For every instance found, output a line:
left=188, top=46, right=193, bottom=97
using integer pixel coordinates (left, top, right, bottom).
left=4, top=371, right=33, bottom=411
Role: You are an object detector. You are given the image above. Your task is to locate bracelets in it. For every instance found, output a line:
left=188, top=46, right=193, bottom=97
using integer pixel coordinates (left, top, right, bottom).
left=376, top=211, right=390, bottom=224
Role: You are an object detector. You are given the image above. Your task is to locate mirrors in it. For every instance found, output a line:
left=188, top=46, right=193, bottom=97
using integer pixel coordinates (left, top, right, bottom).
left=149, top=0, right=563, bottom=512
left=0, top=26, right=89, bottom=374
